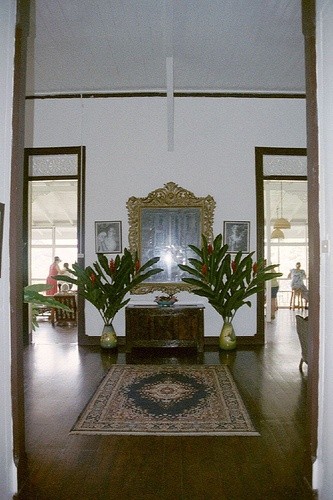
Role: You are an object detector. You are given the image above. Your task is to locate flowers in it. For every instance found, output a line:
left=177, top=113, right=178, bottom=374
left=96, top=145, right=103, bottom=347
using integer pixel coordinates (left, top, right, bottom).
left=178, top=234, right=283, bottom=322
left=51, top=248, right=164, bottom=324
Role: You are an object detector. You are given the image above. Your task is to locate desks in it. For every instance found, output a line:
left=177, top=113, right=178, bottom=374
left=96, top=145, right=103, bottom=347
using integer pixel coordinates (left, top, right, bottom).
left=124, top=304, right=205, bottom=362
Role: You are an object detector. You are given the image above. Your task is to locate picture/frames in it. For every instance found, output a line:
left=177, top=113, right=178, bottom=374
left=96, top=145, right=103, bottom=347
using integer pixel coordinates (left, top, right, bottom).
left=222, top=220, right=251, bottom=254
left=94, top=220, right=122, bottom=254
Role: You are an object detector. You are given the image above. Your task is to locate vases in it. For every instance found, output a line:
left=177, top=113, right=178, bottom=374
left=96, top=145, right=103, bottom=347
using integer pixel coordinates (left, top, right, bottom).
left=100, top=324, right=118, bottom=349
left=220, top=322, right=237, bottom=350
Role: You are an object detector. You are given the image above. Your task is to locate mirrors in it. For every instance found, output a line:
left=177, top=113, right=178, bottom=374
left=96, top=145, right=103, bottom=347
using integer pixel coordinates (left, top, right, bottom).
left=125, top=181, right=217, bottom=296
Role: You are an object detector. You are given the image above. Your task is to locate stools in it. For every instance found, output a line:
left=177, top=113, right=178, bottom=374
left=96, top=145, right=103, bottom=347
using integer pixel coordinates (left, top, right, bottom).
left=289, top=289, right=303, bottom=311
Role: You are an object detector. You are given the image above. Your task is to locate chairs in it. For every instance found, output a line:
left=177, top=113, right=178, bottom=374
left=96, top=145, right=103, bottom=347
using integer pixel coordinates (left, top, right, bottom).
left=51, top=295, right=77, bottom=326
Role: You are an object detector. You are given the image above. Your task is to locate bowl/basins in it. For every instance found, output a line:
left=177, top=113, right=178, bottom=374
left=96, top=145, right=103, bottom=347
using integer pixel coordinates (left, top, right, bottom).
left=156, top=301, right=176, bottom=305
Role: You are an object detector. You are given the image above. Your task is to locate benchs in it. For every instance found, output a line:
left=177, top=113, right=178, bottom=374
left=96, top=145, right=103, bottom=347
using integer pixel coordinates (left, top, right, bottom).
left=296, top=315, right=309, bottom=370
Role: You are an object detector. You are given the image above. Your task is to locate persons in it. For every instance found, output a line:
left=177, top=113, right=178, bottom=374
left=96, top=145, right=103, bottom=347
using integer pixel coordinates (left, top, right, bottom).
left=45, top=257, right=73, bottom=321
left=271, top=261, right=279, bottom=319
left=227, top=225, right=246, bottom=250
left=97, top=227, right=118, bottom=250
left=288, top=262, right=307, bottom=310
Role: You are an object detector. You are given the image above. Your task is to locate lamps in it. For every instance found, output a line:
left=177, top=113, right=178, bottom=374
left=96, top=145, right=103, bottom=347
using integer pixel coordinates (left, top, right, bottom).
left=272, top=206, right=285, bottom=239
left=274, top=182, right=291, bottom=230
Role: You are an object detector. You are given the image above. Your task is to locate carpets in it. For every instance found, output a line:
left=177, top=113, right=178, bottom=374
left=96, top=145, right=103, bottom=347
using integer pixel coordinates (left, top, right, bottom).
left=69, top=362, right=261, bottom=437
